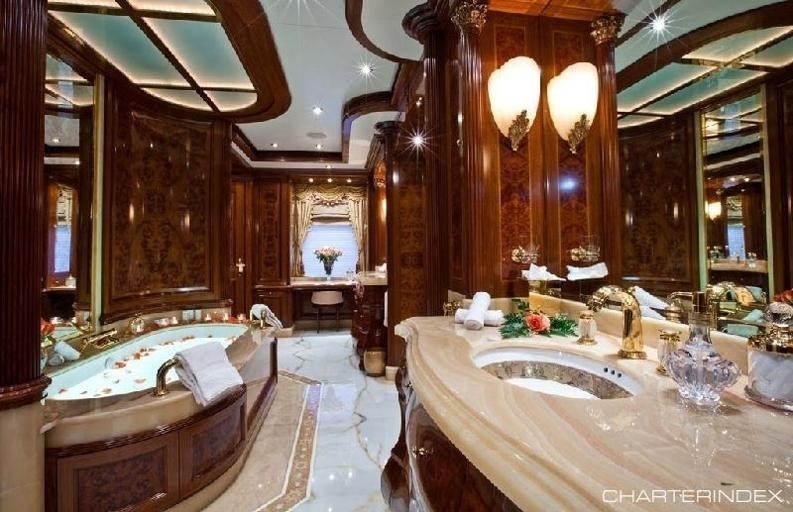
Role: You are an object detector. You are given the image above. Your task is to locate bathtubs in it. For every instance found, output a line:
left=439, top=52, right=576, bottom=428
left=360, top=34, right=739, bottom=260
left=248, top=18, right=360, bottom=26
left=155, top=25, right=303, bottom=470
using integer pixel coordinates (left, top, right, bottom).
left=43, top=323, right=250, bottom=401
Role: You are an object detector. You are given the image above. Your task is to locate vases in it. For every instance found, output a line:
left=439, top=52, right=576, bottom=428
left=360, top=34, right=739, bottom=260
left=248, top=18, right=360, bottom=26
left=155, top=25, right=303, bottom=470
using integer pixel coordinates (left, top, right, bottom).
left=324, top=261, right=334, bottom=280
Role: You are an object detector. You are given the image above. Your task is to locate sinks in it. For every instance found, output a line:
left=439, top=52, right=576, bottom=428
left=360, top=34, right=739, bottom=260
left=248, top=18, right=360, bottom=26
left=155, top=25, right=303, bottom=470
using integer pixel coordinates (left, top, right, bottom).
left=468, top=342, right=642, bottom=399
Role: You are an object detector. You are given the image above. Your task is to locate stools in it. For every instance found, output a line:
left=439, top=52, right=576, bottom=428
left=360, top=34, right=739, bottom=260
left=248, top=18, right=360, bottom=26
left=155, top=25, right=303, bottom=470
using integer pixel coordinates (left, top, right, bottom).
left=310, top=290, right=343, bottom=333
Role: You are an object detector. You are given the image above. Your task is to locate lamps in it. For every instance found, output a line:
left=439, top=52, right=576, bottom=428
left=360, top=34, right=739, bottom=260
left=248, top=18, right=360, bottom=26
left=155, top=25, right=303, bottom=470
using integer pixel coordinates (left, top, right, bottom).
left=546, top=62, right=600, bottom=153
left=487, top=56, right=542, bottom=149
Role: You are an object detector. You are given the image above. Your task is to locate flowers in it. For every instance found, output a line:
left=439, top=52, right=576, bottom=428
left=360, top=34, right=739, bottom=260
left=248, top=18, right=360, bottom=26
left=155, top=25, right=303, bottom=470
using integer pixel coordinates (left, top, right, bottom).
left=499, top=299, right=579, bottom=339
left=313, top=246, right=344, bottom=264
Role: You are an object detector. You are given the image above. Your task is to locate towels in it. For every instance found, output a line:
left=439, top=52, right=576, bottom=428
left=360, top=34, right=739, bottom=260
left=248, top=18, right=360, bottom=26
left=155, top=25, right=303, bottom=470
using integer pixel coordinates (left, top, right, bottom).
left=634, top=285, right=669, bottom=310
left=251, top=302, right=284, bottom=331
left=48, top=353, right=65, bottom=367
left=53, top=341, right=81, bottom=360
left=464, top=292, right=491, bottom=329
left=453, top=308, right=506, bottom=327
left=639, top=303, right=666, bottom=319
left=173, top=343, right=245, bottom=410
left=566, top=261, right=609, bottom=282
left=523, top=263, right=566, bottom=283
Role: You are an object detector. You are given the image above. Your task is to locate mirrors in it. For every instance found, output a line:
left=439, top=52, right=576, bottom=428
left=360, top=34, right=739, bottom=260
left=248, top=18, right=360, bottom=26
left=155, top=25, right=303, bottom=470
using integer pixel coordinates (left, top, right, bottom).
left=521, top=1, right=792, bottom=342
left=693, top=84, right=773, bottom=303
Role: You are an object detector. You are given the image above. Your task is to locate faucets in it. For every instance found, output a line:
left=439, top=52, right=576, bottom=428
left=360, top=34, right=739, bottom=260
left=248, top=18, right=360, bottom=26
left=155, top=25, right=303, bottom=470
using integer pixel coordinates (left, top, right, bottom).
left=587, top=285, right=647, bottom=359
left=156, top=359, right=179, bottom=395
left=706, top=280, right=762, bottom=331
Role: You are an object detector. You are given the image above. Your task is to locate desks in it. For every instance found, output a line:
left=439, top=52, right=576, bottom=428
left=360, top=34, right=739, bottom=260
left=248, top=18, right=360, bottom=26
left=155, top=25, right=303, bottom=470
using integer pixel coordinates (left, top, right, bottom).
left=290, top=280, right=388, bottom=292
